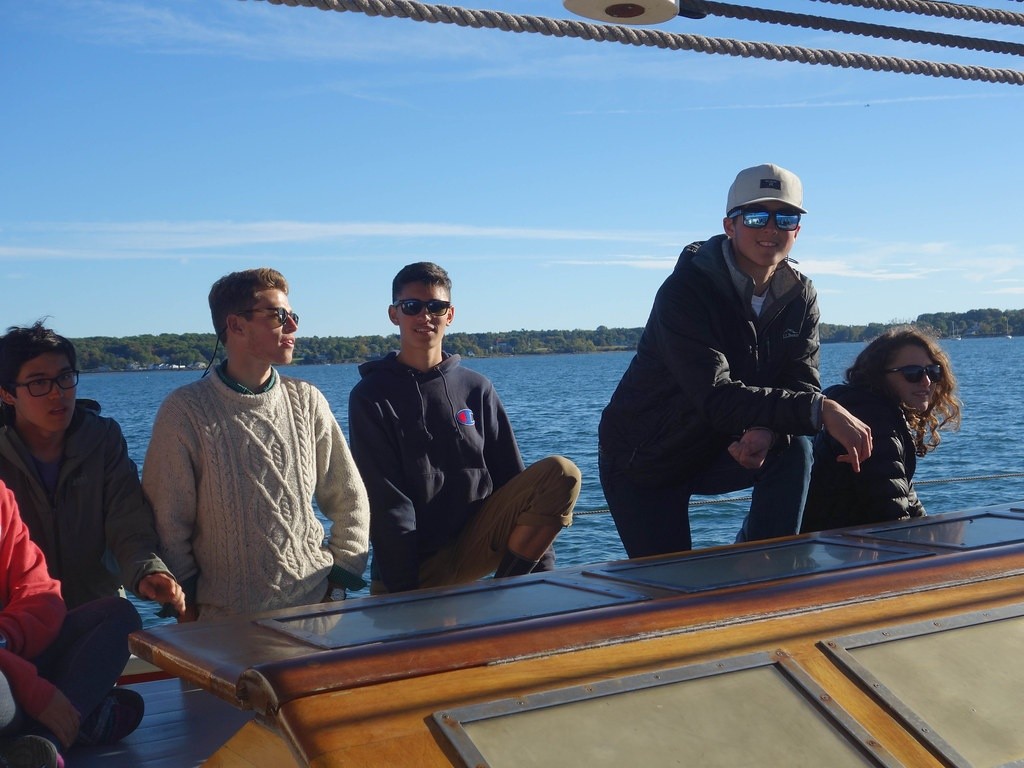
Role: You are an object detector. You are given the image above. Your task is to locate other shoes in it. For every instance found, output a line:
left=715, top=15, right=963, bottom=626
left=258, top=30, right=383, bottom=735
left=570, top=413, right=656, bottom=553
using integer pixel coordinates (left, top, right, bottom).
left=72, top=687, right=145, bottom=748
left=0, top=733, right=64, bottom=768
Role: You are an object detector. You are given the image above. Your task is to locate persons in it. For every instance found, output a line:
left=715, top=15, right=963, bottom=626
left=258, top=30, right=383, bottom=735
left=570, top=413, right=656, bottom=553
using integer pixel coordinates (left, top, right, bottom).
left=139, top=268, right=371, bottom=625
left=799, top=323, right=963, bottom=534
left=348, top=262, right=583, bottom=595
left=596, top=164, right=874, bottom=559
left=0, top=323, right=188, bottom=768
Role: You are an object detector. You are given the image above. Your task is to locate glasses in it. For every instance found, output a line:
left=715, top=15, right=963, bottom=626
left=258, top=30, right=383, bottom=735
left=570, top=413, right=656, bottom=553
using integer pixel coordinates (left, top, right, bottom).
left=6, top=369, right=81, bottom=397
left=393, top=298, right=451, bottom=316
left=236, top=308, right=300, bottom=325
left=884, top=363, right=943, bottom=384
left=729, top=208, right=801, bottom=232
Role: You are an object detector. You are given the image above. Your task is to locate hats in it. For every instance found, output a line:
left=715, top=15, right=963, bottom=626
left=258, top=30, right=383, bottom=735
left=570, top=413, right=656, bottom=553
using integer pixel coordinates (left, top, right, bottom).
left=726, top=164, right=808, bottom=217
